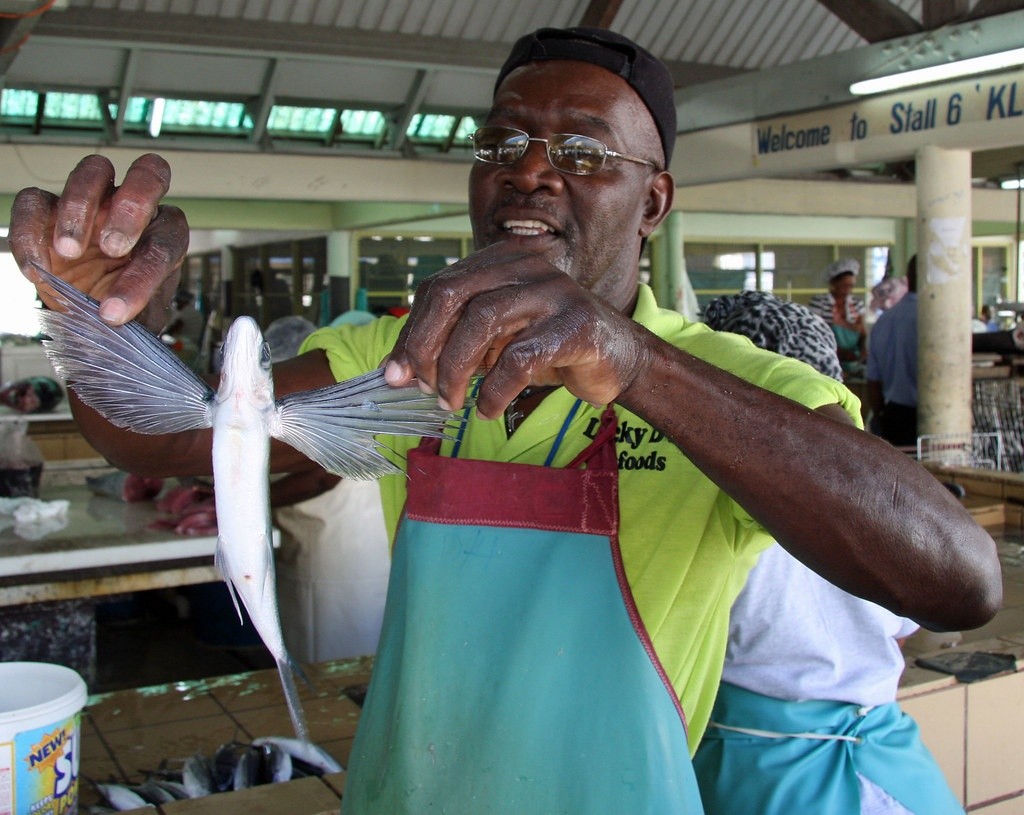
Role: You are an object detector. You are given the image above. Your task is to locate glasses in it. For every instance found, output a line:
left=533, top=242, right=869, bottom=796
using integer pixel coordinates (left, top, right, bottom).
left=468, top=125, right=657, bottom=176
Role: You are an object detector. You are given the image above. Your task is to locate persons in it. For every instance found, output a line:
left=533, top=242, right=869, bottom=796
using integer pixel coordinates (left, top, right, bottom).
left=248, top=267, right=293, bottom=325
left=979, top=304, right=1000, bottom=333
left=693, top=291, right=967, bottom=815
left=158, top=289, right=203, bottom=364
left=8, top=27, right=1004, bottom=814
left=805, top=256, right=867, bottom=380
left=867, top=253, right=918, bottom=443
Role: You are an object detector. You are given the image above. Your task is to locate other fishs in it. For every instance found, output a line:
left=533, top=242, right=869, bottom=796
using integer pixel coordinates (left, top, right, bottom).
left=0, top=375, right=67, bottom=414
left=87, top=740, right=292, bottom=813
left=26, top=257, right=494, bottom=775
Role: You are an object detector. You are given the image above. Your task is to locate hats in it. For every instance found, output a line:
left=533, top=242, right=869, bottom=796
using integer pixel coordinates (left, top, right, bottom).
left=821, top=257, right=861, bottom=281
left=493, top=26, right=678, bottom=172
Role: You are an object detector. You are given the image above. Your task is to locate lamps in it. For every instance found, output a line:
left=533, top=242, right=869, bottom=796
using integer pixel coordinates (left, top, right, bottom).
left=849, top=47, right=1024, bottom=95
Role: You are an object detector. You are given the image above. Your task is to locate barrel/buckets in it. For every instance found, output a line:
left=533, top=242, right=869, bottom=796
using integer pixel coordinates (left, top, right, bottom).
left=1, top=661, right=88, bottom=815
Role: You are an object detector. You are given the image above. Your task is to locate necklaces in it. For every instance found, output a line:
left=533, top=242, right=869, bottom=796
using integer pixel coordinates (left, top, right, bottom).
left=505, top=384, right=556, bottom=433
